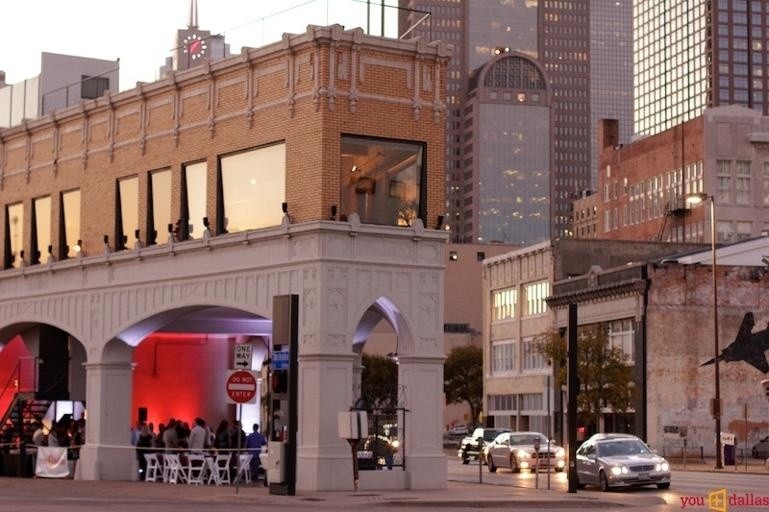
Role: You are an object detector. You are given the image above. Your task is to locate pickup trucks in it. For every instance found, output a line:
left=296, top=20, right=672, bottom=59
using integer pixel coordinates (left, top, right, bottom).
left=457, top=427, right=513, bottom=466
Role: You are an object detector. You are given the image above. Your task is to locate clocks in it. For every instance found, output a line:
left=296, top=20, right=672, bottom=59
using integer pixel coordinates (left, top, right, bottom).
left=183, top=33, right=207, bottom=60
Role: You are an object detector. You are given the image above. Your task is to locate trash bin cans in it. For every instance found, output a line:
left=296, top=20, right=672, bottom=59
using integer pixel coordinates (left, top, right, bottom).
left=724, top=445, right=735, bottom=465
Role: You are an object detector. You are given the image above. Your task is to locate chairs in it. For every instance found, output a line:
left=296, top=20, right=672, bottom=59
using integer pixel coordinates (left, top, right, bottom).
left=143, top=454, right=254, bottom=486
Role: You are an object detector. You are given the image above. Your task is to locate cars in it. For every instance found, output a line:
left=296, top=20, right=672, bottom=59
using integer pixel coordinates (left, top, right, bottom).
left=483, top=430, right=565, bottom=474
left=385, top=427, right=397, bottom=447
left=751, top=435, right=769, bottom=459
left=576, top=431, right=671, bottom=492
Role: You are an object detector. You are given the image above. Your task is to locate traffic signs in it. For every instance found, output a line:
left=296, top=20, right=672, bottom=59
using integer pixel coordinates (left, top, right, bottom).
left=233, top=344, right=252, bottom=370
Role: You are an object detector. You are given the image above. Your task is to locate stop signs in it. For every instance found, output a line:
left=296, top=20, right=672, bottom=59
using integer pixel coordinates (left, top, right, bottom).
left=224, top=369, right=257, bottom=404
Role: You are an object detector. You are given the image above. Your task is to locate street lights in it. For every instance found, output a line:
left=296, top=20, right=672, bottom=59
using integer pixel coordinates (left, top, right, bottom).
left=684, top=194, right=726, bottom=470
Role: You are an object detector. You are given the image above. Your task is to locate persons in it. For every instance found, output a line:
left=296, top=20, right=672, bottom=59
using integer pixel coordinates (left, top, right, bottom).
left=32, top=412, right=89, bottom=447
left=128, top=416, right=265, bottom=481
